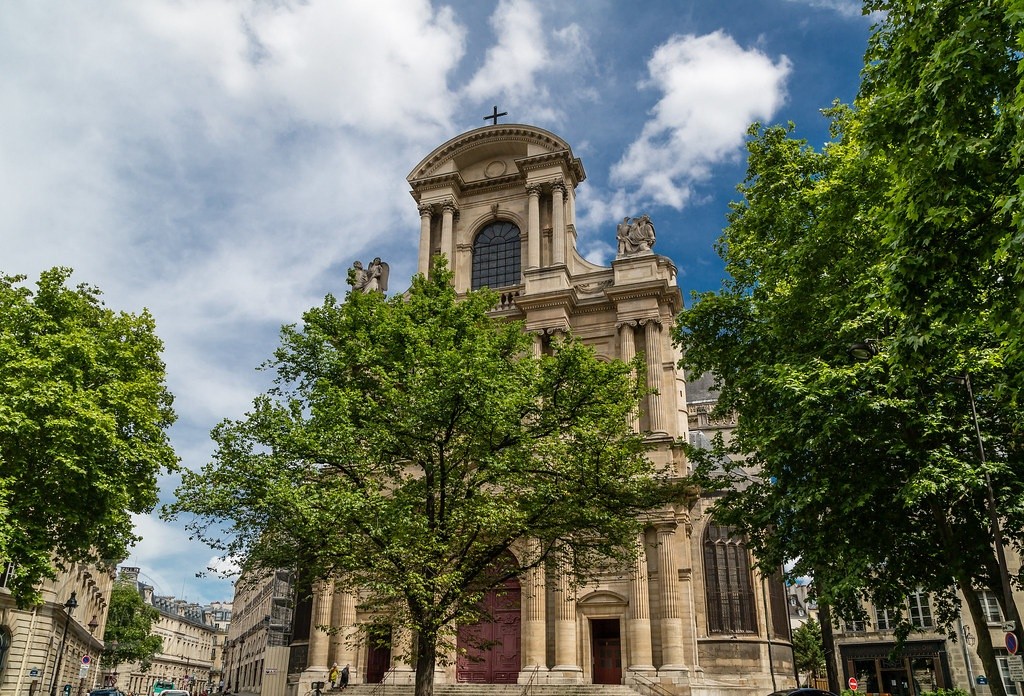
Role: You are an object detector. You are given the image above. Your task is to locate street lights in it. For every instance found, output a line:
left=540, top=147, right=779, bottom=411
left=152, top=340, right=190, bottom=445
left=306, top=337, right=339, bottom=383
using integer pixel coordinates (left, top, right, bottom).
left=50, top=590, right=80, bottom=696
left=77, top=615, right=98, bottom=696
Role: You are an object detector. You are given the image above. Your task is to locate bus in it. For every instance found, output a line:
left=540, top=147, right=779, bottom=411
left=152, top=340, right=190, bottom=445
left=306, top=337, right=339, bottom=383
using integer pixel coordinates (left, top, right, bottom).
left=153, top=680, right=174, bottom=696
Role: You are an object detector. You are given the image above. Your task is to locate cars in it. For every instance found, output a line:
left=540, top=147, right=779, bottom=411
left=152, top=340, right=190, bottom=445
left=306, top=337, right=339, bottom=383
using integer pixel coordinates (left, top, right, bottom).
left=158, top=690, right=190, bottom=696
left=767, top=688, right=838, bottom=696
left=89, top=689, right=127, bottom=696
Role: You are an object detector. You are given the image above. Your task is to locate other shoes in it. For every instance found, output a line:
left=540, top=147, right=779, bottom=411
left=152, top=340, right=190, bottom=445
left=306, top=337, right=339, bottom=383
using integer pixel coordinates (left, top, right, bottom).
left=340, top=687, right=342, bottom=691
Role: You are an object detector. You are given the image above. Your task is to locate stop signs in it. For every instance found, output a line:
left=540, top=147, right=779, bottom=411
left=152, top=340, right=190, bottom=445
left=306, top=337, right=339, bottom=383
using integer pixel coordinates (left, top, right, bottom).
left=849, top=677, right=858, bottom=691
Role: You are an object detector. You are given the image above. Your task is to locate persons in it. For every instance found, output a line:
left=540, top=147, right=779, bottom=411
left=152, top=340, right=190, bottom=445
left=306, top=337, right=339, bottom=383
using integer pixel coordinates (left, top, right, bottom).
left=212, top=678, right=224, bottom=693
left=85, top=690, right=90, bottom=696
left=351, top=257, right=382, bottom=294
left=626, top=214, right=654, bottom=252
left=128, top=691, right=139, bottom=696
left=329, top=663, right=339, bottom=691
left=339, top=663, right=350, bottom=692
